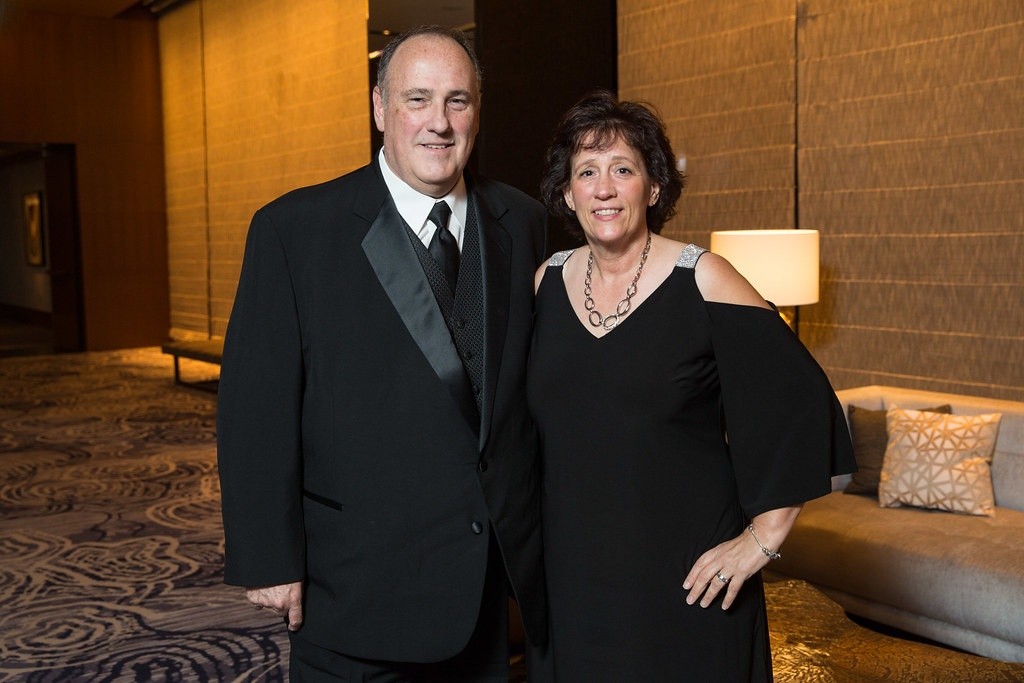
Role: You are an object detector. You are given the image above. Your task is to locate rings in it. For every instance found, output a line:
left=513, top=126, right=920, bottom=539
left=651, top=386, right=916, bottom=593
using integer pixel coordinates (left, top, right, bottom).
left=717, top=573, right=728, bottom=584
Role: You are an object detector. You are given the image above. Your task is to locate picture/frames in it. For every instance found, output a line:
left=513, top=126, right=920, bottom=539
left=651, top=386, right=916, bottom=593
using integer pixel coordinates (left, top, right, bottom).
left=21, top=190, right=46, bottom=267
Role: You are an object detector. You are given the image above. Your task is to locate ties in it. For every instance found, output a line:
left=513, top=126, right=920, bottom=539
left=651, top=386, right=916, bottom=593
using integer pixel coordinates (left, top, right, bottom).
left=427, top=200, right=461, bottom=299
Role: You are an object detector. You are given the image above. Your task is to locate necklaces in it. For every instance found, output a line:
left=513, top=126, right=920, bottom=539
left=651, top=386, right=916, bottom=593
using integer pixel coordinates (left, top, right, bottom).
left=584, top=231, right=651, bottom=330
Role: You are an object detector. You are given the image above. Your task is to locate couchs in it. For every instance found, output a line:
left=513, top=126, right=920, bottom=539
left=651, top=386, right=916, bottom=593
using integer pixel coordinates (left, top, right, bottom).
left=762, top=384, right=1024, bottom=665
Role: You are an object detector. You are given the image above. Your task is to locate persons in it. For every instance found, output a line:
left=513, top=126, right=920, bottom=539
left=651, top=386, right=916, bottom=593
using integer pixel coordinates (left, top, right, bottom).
left=524, top=91, right=858, bottom=682
left=216, top=27, right=731, bottom=683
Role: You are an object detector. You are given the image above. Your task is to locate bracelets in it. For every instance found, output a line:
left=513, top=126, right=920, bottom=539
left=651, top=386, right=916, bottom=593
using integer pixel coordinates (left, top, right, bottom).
left=747, top=525, right=781, bottom=560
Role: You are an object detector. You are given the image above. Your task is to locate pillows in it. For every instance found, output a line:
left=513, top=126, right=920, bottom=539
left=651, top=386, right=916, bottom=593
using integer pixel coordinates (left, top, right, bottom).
left=878, top=402, right=1003, bottom=519
left=842, top=403, right=952, bottom=495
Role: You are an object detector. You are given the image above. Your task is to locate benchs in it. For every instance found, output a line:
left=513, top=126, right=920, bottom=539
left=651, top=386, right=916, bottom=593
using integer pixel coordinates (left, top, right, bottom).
left=161, top=339, right=224, bottom=394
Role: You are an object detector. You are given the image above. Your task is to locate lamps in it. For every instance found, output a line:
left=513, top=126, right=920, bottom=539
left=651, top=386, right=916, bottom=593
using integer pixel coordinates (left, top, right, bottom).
left=709, top=228, right=820, bottom=337
